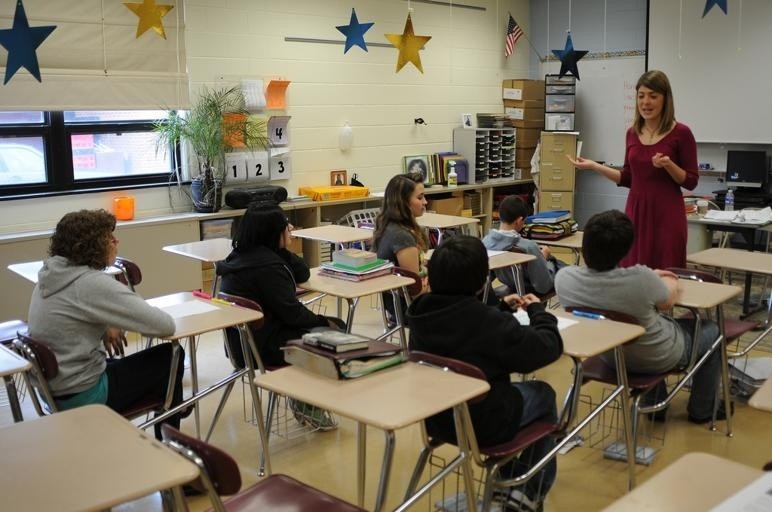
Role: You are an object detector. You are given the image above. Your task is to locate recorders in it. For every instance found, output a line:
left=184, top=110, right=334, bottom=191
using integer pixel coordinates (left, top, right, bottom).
left=225, top=185, right=287, bottom=209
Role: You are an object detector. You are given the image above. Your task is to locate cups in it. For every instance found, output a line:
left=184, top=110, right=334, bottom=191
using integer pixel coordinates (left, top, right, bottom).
left=696, top=201, right=708, bottom=215
left=112, top=196, right=135, bottom=222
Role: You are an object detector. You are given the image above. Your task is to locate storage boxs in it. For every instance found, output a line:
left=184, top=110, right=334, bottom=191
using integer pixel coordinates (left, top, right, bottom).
left=546, top=113, right=576, bottom=131
left=502, top=99, right=545, bottom=120
left=516, top=147, right=540, bottom=162
left=502, top=77, right=545, bottom=102
left=544, top=70, right=577, bottom=96
left=509, top=118, right=545, bottom=145
left=515, top=160, right=532, bottom=179
left=546, top=95, right=579, bottom=115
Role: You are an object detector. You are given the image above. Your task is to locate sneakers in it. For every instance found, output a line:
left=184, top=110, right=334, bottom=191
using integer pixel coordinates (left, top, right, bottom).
left=689, top=401, right=735, bottom=423
left=492, top=486, right=543, bottom=512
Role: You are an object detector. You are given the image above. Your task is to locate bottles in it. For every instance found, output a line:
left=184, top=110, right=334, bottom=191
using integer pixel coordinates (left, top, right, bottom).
left=724, top=189, right=736, bottom=219
left=447, top=167, right=458, bottom=189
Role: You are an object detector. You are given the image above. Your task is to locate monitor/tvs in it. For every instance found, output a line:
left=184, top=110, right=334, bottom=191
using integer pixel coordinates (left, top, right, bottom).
left=726, top=149, right=767, bottom=188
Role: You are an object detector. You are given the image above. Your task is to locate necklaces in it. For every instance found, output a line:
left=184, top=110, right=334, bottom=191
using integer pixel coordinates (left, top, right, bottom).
left=647, top=127, right=658, bottom=142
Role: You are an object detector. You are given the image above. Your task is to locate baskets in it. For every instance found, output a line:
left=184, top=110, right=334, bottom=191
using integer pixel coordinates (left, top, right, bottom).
left=337, top=207, right=381, bottom=228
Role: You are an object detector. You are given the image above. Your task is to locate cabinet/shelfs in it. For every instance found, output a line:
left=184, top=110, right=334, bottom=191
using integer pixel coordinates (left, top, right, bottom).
left=451, top=124, right=518, bottom=185
left=385, top=178, right=489, bottom=243
left=535, top=129, right=578, bottom=226
left=484, top=179, right=535, bottom=230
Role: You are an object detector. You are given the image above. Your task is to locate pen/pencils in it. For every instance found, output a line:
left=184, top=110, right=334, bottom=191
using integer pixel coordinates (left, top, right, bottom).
left=572, top=309, right=610, bottom=321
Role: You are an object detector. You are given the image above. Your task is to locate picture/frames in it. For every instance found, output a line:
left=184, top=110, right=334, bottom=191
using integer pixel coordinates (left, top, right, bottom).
left=403, top=154, right=435, bottom=189
left=330, top=169, right=349, bottom=187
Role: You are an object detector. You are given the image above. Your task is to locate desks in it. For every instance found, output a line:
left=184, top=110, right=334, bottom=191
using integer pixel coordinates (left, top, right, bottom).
left=0, top=201, right=772, bottom=512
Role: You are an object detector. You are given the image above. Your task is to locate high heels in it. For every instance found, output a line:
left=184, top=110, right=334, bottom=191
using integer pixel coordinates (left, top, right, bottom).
left=290, top=397, right=339, bottom=430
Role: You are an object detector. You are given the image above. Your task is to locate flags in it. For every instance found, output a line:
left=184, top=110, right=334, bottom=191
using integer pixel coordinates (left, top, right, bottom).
left=505, top=16, right=523, bottom=57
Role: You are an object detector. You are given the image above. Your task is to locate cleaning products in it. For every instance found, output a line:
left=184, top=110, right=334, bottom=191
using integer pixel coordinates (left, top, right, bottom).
left=447, top=159, right=459, bottom=188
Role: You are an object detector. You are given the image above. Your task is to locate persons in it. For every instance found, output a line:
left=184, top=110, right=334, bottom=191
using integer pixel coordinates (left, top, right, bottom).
left=565, top=69, right=699, bottom=270
left=28, top=209, right=202, bottom=496
left=481, top=192, right=569, bottom=297
left=401, top=236, right=557, bottom=511
left=554, top=209, right=734, bottom=424
left=218, top=202, right=343, bottom=432
left=372, top=171, right=500, bottom=330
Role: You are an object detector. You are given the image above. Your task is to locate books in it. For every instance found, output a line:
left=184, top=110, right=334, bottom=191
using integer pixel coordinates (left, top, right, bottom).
left=320, top=248, right=394, bottom=282
left=602, top=442, right=660, bottom=464
left=432, top=151, right=466, bottom=185
left=301, top=330, right=370, bottom=353
left=526, top=211, right=571, bottom=224
left=433, top=492, right=502, bottom=511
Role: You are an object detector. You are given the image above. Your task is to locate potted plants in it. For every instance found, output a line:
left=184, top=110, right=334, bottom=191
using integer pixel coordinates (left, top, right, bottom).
left=149, top=78, right=281, bottom=216
